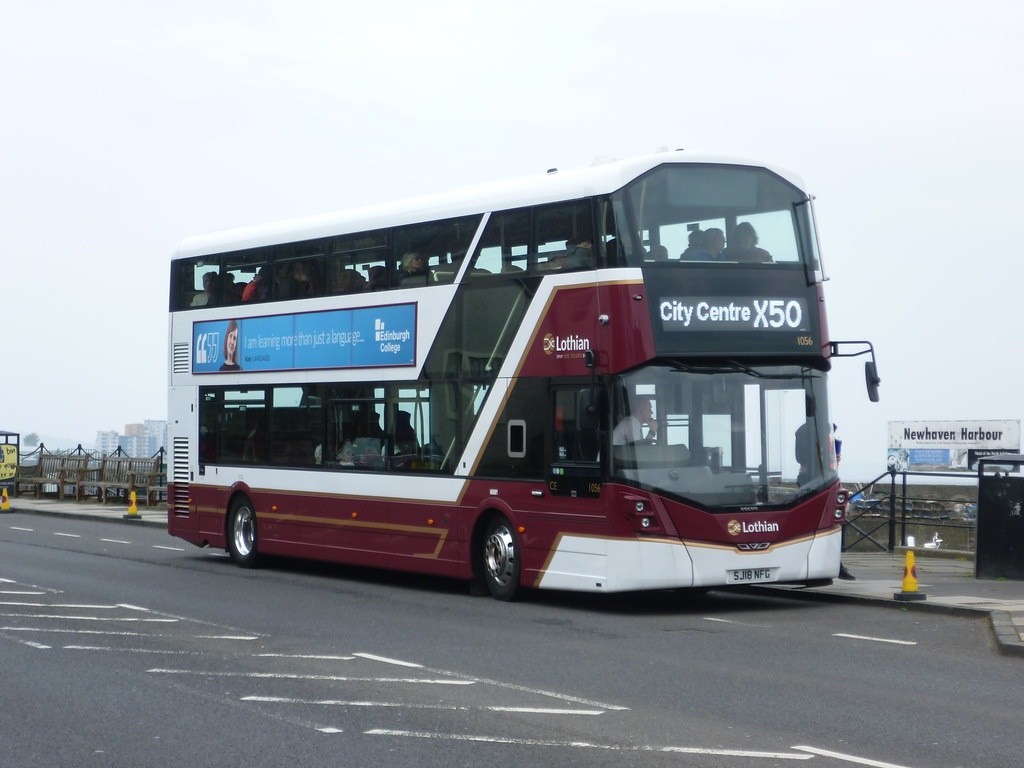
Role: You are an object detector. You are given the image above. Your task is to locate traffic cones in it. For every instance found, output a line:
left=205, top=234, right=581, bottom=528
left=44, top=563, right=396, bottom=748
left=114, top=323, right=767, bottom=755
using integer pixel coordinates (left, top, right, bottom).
left=893, top=550, right=926, bottom=600
left=124, top=491, right=140, bottom=520
left=0, top=487, right=14, bottom=513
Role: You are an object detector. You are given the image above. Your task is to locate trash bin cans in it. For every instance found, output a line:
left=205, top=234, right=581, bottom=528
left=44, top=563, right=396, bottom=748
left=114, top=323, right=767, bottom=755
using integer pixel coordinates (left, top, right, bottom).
left=973, top=454, right=1024, bottom=581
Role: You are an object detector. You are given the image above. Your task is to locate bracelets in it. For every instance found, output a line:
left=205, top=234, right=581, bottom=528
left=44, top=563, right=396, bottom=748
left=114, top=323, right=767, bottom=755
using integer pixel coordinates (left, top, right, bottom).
left=649, top=430, right=656, bottom=436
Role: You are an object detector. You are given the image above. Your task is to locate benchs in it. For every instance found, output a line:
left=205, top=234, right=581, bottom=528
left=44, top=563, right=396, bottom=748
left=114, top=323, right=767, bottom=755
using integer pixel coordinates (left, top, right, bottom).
left=12, top=454, right=167, bottom=508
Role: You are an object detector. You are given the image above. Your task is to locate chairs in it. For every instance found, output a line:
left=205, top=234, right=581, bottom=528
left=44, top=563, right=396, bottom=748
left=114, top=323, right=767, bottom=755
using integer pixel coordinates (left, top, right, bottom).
left=198, top=227, right=752, bottom=470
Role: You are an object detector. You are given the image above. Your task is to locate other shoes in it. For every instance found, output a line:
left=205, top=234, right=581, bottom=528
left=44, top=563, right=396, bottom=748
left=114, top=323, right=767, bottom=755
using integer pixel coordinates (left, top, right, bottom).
left=838, top=564, right=856, bottom=581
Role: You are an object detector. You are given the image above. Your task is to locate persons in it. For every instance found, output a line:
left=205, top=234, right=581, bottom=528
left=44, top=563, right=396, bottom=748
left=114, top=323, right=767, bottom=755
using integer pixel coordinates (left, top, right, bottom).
left=395, top=250, right=426, bottom=286
left=795, top=420, right=855, bottom=579
left=693, top=228, right=725, bottom=261
left=188, top=271, right=217, bottom=306
left=721, top=222, right=772, bottom=261
left=595, top=397, right=658, bottom=462
left=679, top=230, right=703, bottom=259
left=226, top=260, right=319, bottom=308
left=649, top=245, right=667, bottom=260
left=559, top=237, right=617, bottom=268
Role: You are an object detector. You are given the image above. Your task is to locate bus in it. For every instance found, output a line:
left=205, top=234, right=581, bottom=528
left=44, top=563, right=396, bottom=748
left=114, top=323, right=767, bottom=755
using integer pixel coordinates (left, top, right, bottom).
left=166, top=149, right=880, bottom=600
